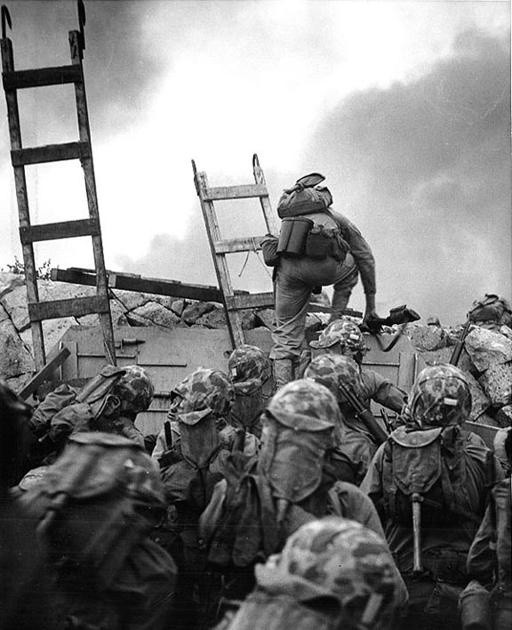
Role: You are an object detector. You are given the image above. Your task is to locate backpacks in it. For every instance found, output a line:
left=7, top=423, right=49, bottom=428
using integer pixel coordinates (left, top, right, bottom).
left=466, top=294, right=512, bottom=328
left=276, top=172, right=333, bottom=217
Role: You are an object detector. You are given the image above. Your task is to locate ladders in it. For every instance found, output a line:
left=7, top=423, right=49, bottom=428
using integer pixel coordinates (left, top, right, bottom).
left=192, top=152, right=282, bottom=348
left=1, top=29, right=118, bottom=399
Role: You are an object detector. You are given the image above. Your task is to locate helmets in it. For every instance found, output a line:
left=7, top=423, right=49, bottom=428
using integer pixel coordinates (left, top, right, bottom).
left=113, top=365, right=155, bottom=414
left=115, top=452, right=165, bottom=501
left=279, top=515, right=406, bottom=615
left=180, top=318, right=366, bottom=451
left=406, top=362, right=472, bottom=426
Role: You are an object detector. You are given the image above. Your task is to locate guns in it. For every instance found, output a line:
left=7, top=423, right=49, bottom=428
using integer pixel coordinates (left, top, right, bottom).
left=380, top=408, right=394, bottom=433
left=358, top=305, right=420, bottom=330
left=338, top=377, right=389, bottom=447
left=449, top=320, right=471, bottom=365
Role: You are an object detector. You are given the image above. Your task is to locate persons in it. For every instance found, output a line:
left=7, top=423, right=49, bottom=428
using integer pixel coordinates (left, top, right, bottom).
left=267, top=202, right=384, bottom=396
left=1, top=290, right=511, bottom=630
